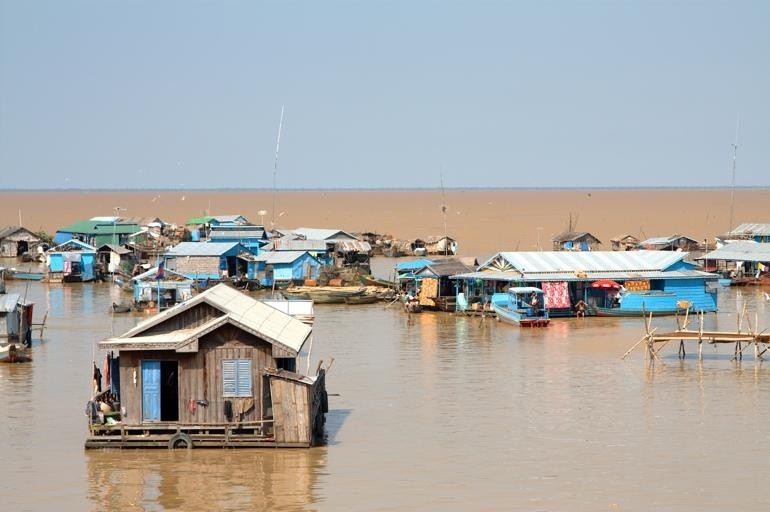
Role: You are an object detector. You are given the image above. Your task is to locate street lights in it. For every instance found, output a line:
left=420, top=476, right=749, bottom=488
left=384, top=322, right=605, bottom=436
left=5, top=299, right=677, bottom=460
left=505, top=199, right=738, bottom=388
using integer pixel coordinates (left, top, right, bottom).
left=731, top=140, right=738, bottom=233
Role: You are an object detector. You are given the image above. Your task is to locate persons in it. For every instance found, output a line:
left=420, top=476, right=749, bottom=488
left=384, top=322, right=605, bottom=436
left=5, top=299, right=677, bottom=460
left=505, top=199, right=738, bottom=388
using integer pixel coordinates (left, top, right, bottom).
left=612, top=288, right=622, bottom=309
left=528, top=291, right=541, bottom=315
left=574, top=299, right=589, bottom=319
left=398, top=288, right=422, bottom=313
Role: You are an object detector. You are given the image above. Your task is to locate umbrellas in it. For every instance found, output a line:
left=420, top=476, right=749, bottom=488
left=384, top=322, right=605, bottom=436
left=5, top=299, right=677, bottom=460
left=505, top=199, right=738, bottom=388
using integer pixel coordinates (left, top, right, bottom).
left=592, top=280, right=620, bottom=309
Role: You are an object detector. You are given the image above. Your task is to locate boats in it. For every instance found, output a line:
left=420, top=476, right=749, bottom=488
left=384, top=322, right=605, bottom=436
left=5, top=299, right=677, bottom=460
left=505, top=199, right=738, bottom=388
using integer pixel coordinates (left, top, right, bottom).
left=718, top=277, right=749, bottom=286
left=0, top=267, right=45, bottom=281
left=750, top=275, right=770, bottom=286
left=113, top=301, right=130, bottom=313
left=279, top=283, right=399, bottom=305
left=1, top=341, right=32, bottom=364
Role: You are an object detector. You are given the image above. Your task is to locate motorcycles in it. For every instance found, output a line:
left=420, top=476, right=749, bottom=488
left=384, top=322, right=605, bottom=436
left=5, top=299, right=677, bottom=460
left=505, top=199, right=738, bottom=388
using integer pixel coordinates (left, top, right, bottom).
left=491, top=285, right=551, bottom=328
